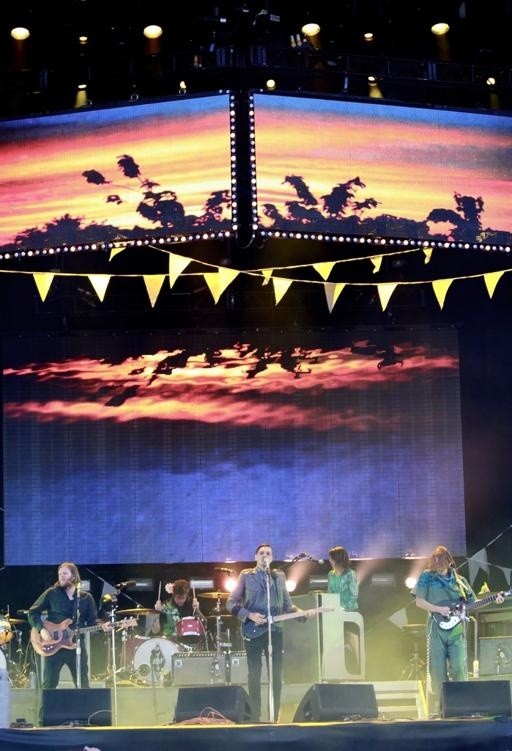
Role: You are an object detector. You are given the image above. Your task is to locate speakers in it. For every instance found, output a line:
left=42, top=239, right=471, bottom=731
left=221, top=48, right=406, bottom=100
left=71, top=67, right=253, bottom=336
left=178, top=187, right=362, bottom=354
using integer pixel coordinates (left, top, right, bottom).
left=439, top=679, right=512, bottom=718
left=41, top=687, right=112, bottom=727
left=174, top=685, right=258, bottom=723
left=293, top=683, right=380, bottom=720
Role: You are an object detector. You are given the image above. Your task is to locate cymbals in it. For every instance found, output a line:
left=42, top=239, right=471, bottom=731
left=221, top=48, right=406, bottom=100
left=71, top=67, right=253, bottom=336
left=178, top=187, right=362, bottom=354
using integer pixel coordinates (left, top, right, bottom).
left=197, top=592, right=231, bottom=599
left=208, top=614, right=233, bottom=622
left=116, top=609, right=160, bottom=615
left=2, top=618, right=24, bottom=625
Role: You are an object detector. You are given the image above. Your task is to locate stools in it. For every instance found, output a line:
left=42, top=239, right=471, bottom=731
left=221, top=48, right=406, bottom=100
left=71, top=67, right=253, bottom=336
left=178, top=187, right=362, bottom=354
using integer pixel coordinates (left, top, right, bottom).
left=401, top=623, right=427, bottom=680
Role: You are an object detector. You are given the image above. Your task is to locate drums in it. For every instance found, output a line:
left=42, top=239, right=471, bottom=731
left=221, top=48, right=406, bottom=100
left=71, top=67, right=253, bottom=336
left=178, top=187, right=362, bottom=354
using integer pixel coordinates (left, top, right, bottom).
left=0, top=618, right=14, bottom=644
left=176, top=616, right=202, bottom=644
left=132, top=638, right=181, bottom=686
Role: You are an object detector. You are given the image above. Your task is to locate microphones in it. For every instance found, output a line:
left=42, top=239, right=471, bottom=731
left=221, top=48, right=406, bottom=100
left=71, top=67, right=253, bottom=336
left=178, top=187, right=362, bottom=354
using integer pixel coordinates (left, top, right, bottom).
left=309, top=558, right=324, bottom=564
left=116, top=579, right=136, bottom=588
left=263, top=557, right=272, bottom=574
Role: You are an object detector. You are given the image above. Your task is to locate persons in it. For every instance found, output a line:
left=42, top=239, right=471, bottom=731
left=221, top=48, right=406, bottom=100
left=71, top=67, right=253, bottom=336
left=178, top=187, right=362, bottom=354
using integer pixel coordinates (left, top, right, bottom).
left=226, top=544, right=315, bottom=723
left=328, top=546, right=359, bottom=673
left=154, top=579, right=200, bottom=644
left=415, top=546, right=505, bottom=720
left=27, top=562, right=112, bottom=727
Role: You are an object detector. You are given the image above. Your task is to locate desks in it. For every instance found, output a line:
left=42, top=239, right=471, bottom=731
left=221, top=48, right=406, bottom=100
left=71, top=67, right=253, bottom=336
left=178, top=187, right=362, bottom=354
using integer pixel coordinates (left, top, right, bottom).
left=468, top=605, right=512, bottom=676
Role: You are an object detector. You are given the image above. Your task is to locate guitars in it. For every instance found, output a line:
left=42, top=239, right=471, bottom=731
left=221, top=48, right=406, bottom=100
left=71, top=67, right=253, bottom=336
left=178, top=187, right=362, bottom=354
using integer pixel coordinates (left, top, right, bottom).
left=243, top=607, right=335, bottom=638
left=431, top=587, right=512, bottom=630
left=31, top=616, right=138, bottom=657
